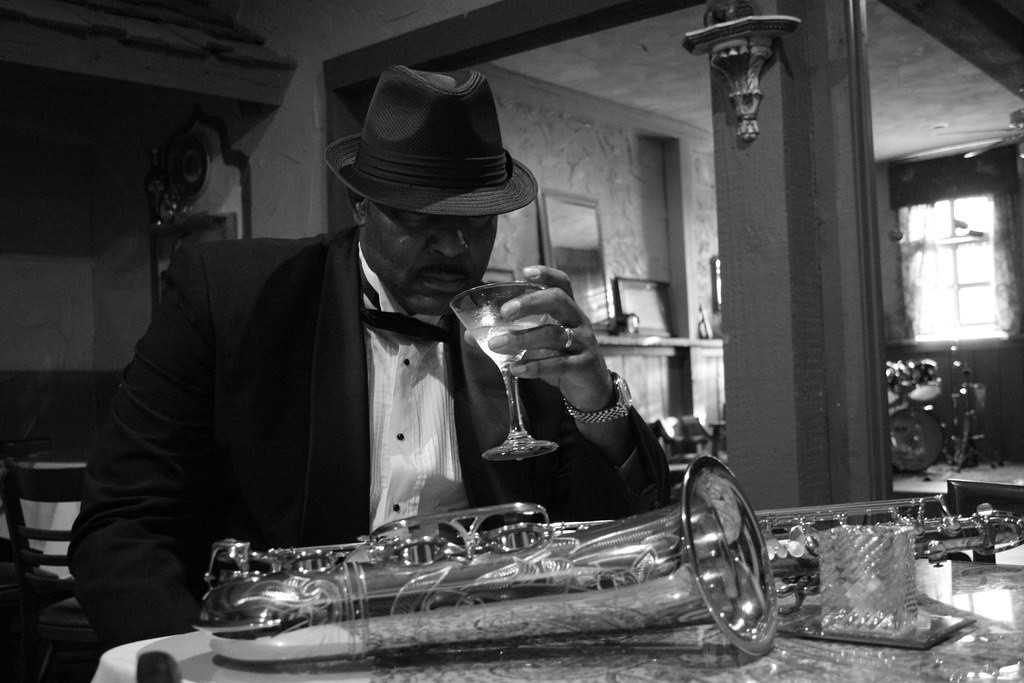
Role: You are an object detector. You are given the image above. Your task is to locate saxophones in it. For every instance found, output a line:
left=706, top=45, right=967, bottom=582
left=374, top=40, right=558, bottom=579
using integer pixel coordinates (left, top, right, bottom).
left=191, top=453, right=1024, bottom=662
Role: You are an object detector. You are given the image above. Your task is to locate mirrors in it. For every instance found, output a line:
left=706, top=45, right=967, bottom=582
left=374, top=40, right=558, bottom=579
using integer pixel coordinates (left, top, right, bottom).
left=537, top=186, right=614, bottom=335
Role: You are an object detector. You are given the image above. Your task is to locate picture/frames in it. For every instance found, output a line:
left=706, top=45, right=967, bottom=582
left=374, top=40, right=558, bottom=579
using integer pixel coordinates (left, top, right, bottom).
left=614, top=277, right=677, bottom=337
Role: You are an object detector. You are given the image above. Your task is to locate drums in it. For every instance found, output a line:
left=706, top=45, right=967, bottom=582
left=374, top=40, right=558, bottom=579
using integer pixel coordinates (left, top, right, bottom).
left=886, top=359, right=944, bottom=475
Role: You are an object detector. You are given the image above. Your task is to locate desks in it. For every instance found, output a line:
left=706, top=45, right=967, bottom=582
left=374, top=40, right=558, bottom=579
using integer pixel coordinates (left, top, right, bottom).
left=89, top=543, right=1024, bottom=683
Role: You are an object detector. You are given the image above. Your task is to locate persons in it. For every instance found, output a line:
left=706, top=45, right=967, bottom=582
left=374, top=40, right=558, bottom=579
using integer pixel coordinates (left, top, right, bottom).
left=66, top=65, right=672, bottom=652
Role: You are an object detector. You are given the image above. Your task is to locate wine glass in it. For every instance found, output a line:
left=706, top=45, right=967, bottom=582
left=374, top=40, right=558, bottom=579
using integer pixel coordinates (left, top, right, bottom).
left=449, top=281, right=559, bottom=460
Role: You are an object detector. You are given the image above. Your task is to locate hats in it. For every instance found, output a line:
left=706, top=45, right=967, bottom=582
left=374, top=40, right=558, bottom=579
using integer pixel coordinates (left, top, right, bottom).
left=323, top=63, right=538, bottom=217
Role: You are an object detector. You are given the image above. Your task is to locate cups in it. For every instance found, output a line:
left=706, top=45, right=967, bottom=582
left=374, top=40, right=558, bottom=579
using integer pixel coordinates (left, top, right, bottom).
left=158, top=197, right=192, bottom=224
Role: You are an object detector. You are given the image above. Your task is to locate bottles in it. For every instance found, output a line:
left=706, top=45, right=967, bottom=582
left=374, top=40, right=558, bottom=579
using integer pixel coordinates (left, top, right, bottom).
left=144, top=149, right=169, bottom=225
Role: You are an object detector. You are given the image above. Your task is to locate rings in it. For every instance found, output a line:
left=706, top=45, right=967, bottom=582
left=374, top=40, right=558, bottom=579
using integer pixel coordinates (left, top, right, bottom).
left=561, top=328, right=574, bottom=353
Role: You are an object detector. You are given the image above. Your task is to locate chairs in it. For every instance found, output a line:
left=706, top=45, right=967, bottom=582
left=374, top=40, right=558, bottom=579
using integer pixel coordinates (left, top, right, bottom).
left=0, top=460, right=104, bottom=683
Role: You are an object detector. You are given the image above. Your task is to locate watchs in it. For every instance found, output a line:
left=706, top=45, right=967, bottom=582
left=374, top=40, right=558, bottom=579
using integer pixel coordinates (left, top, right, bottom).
left=563, top=372, right=632, bottom=424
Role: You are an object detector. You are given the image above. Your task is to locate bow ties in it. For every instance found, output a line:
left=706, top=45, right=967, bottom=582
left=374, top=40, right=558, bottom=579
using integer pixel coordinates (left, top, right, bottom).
left=358, top=263, right=461, bottom=343
